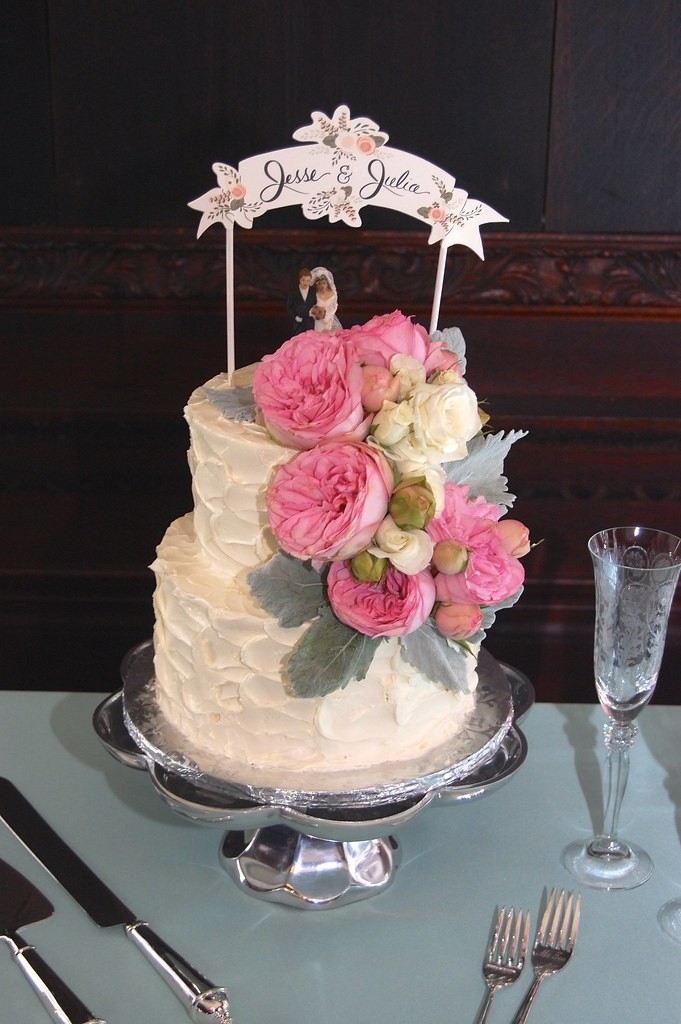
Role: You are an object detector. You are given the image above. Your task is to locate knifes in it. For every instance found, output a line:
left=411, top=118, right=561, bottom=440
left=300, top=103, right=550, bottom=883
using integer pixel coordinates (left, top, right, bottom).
left=0, top=858, right=108, bottom=1024
left=0, top=776, right=233, bottom=1024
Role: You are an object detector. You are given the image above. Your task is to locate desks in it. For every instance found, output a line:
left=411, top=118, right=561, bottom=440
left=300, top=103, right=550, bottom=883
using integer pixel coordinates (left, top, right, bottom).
left=0, top=687, right=681, bottom=1024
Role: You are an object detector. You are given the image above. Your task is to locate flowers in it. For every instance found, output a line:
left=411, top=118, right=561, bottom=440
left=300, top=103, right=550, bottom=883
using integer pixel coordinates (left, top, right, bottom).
left=247, top=309, right=543, bottom=696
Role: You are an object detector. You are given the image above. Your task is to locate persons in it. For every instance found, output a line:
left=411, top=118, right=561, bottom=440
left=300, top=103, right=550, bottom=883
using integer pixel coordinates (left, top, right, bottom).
left=286, top=266, right=343, bottom=340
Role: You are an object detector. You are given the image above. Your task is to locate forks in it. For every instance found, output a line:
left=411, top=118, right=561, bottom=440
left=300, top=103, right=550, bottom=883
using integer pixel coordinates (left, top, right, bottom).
left=512, top=887, right=581, bottom=1024
left=477, top=904, right=531, bottom=1024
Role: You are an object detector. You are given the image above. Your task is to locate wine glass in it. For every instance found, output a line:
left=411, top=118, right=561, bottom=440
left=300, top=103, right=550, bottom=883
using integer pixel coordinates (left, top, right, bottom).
left=560, top=525, right=681, bottom=892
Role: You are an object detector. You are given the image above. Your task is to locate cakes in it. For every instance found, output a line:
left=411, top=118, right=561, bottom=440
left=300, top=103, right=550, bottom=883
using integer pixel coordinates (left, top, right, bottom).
left=147, top=266, right=535, bottom=775
